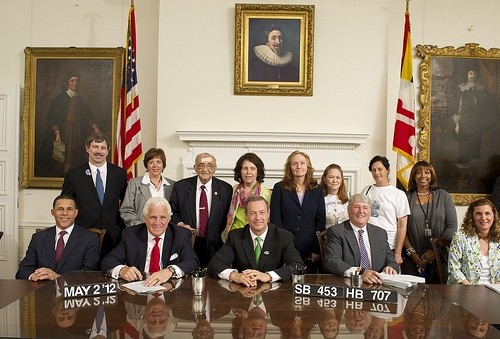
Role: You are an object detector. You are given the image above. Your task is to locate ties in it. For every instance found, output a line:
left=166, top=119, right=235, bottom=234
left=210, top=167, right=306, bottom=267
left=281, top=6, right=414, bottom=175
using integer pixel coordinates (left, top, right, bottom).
left=252, top=293, right=263, bottom=306
left=255, top=237, right=263, bottom=268
left=202, top=279, right=207, bottom=317
left=55, top=231, right=67, bottom=266
left=96, top=306, right=104, bottom=335
left=57, top=276, right=66, bottom=295
left=96, top=169, right=104, bottom=207
left=358, top=230, right=370, bottom=269
left=199, top=186, right=209, bottom=239
left=149, top=238, right=161, bottom=276
left=153, top=293, right=161, bottom=297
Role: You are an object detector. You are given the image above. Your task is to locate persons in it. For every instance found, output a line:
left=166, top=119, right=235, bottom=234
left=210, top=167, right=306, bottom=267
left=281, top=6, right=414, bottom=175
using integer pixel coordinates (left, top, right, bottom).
left=35, top=275, right=492, bottom=339
left=13, top=133, right=500, bottom=285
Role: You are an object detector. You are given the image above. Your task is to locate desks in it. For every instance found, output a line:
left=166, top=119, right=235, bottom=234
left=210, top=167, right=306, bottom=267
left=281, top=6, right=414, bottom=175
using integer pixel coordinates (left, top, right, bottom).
left=0, top=270, right=500, bottom=339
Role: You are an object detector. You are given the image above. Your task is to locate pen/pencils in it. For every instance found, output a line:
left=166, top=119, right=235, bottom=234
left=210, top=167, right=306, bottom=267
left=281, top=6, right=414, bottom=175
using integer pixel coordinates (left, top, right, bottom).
left=290, top=265, right=307, bottom=275
left=192, top=268, right=208, bottom=278
left=351, top=266, right=365, bottom=275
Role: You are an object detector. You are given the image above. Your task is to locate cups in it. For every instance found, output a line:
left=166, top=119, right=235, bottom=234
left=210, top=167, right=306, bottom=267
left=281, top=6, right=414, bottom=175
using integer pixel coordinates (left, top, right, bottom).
left=191, top=274, right=206, bottom=296
left=351, top=274, right=362, bottom=288
left=291, top=273, right=305, bottom=286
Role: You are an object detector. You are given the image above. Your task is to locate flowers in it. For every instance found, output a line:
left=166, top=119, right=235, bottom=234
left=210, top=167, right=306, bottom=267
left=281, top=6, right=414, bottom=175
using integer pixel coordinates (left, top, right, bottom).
left=84, top=169, right=92, bottom=176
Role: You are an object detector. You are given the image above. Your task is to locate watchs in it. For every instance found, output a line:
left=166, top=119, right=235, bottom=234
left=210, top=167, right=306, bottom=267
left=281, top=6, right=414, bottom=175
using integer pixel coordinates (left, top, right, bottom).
left=407, top=248, right=415, bottom=255
left=168, top=266, right=175, bottom=277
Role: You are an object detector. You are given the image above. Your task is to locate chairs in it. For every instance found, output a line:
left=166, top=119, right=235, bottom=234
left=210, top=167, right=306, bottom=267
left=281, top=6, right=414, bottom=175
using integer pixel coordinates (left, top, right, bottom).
left=36, top=228, right=107, bottom=255
left=430, top=237, right=454, bottom=284
left=316, top=230, right=330, bottom=265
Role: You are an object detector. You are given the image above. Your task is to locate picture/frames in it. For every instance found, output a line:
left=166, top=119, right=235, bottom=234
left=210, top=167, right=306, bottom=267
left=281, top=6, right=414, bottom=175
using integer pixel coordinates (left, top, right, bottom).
left=415, top=42, right=500, bottom=206
left=233, top=3, right=315, bottom=97
left=20, top=46, right=126, bottom=189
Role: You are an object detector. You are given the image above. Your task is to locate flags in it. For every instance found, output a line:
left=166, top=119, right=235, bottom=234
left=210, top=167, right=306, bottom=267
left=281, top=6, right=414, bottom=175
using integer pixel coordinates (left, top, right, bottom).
left=115, top=6, right=144, bottom=182
left=392, top=14, right=416, bottom=194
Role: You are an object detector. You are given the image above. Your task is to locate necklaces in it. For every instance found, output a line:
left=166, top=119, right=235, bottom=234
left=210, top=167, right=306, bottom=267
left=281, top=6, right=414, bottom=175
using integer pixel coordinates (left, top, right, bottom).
left=151, top=178, right=162, bottom=188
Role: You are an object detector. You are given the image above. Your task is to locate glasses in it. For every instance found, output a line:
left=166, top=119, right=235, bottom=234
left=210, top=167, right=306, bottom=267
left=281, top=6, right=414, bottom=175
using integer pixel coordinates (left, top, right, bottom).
left=194, top=163, right=216, bottom=170
